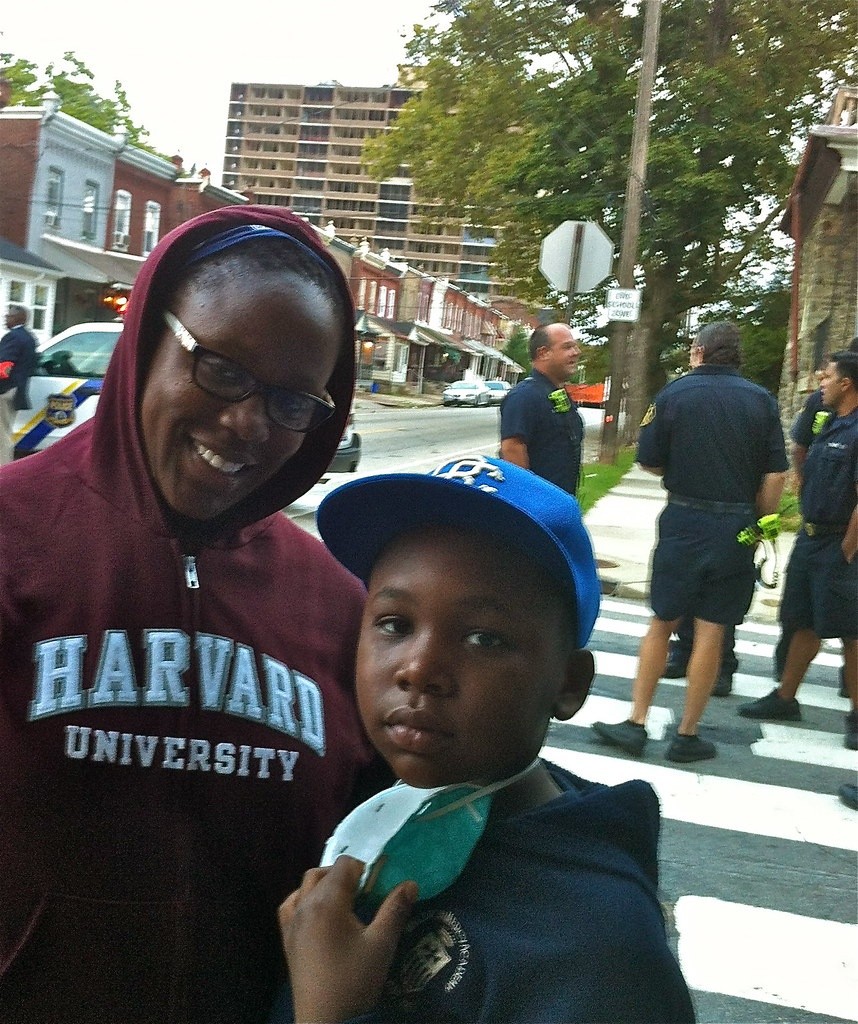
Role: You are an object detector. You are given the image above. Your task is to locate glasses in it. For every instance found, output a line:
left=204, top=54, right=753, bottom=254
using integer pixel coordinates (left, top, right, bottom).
left=159, top=309, right=337, bottom=434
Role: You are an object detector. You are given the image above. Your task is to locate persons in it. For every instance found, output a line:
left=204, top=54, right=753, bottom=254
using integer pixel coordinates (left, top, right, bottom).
left=277, top=454, right=694, bottom=1024
left=0, top=306, right=36, bottom=465
left=499, top=323, right=583, bottom=498
left=0, top=199, right=397, bottom=1024
left=734, top=336, right=858, bottom=752
left=591, top=320, right=791, bottom=762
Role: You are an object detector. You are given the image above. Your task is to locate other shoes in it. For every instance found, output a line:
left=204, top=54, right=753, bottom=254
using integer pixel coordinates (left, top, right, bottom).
left=774, top=649, right=787, bottom=681
left=662, top=654, right=688, bottom=679
left=712, top=672, right=733, bottom=697
left=840, top=665, right=850, bottom=698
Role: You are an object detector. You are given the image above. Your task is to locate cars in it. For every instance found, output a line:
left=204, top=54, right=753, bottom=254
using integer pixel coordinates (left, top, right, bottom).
left=442, top=379, right=490, bottom=408
left=0, top=322, right=366, bottom=485
left=482, top=381, right=512, bottom=406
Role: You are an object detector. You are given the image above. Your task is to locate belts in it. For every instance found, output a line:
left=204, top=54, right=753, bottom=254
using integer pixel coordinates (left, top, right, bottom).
left=667, top=493, right=755, bottom=515
left=802, top=521, right=848, bottom=538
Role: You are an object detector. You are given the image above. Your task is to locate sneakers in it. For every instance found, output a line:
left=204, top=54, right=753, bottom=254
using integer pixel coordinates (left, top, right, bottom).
left=667, top=731, right=715, bottom=763
left=592, top=720, right=645, bottom=755
left=844, top=712, right=858, bottom=752
left=840, top=782, right=858, bottom=810
left=737, top=690, right=801, bottom=720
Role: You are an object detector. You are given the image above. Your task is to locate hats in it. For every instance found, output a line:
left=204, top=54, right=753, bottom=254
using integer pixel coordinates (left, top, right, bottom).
left=317, top=454, right=601, bottom=650
